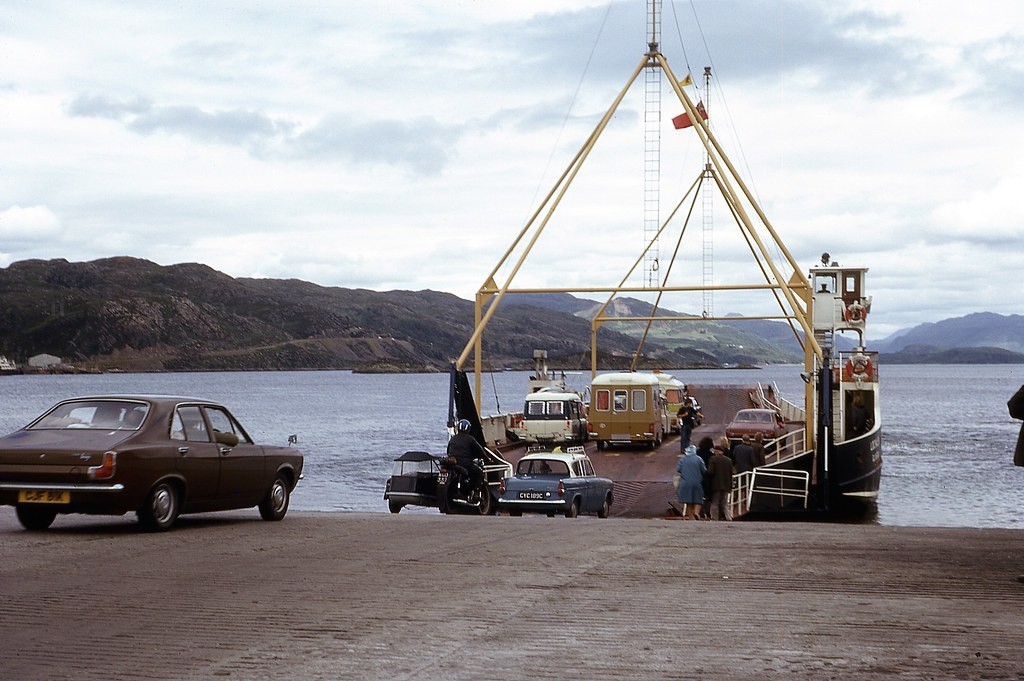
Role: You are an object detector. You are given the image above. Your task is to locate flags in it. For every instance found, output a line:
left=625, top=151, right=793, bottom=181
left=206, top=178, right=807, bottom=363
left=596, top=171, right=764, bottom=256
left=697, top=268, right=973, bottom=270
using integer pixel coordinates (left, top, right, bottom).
left=672, top=101, right=708, bottom=130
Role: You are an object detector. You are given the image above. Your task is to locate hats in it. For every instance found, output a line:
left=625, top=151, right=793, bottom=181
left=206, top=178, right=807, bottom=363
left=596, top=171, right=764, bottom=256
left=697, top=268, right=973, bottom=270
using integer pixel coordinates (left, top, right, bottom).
left=685, top=445, right=696, bottom=453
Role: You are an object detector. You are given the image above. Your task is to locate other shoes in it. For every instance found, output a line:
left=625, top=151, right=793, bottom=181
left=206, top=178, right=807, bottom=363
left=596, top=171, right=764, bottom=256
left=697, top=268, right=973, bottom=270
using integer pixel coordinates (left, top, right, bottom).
left=472, top=495, right=485, bottom=502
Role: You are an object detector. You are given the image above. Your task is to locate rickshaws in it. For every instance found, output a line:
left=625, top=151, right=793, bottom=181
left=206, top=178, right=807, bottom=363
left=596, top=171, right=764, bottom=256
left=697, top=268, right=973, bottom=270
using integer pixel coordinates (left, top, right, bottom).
left=383, top=451, right=493, bottom=515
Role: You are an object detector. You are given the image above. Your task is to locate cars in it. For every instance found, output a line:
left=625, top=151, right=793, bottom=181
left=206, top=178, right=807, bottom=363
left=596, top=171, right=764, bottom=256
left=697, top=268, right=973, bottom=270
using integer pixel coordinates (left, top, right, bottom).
left=725, top=409, right=788, bottom=453
left=0, top=394, right=305, bottom=531
left=683, top=397, right=703, bottom=429
left=497, top=446, right=614, bottom=518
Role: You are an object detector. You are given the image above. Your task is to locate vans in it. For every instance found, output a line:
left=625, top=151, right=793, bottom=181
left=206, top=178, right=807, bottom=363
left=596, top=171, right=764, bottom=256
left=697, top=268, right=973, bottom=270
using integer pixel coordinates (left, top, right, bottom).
left=517, top=387, right=592, bottom=445
left=650, top=373, right=688, bottom=434
left=589, top=372, right=671, bottom=451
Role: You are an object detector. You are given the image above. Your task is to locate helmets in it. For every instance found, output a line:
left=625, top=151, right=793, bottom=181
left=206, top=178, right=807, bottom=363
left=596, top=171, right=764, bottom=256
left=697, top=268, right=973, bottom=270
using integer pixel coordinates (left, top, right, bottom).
left=457, top=419, right=471, bottom=432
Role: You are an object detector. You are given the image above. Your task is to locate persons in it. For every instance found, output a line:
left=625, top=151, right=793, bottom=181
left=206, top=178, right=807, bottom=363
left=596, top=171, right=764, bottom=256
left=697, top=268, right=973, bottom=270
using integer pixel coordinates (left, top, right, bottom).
left=720, top=436, right=732, bottom=460
left=751, top=432, right=766, bottom=467
left=696, top=436, right=714, bottom=519
left=1007, top=384, right=1024, bottom=583
left=849, top=395, right=870, bottom=435
left=125, top=410, right=145, bottom=428
left=676, top=445, right=707, bottom=520
left=447, top=419, right=491, bottom=505
left=178, top=410, right=239, bottom=447
left=733, top=434, right=758, bottom=509
left=702, top=445, right=733, bottom=521
left=818, top=284, right=830, bottom=293
left=677, top=398, right=703, bottom=454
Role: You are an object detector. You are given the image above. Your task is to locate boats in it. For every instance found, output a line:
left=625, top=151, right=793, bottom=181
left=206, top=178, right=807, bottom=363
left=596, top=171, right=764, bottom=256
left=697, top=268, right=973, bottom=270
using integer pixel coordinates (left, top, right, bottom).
left=0, top=356, right=17, bottom=370
left=445, top=0, right=884, bottom=525
